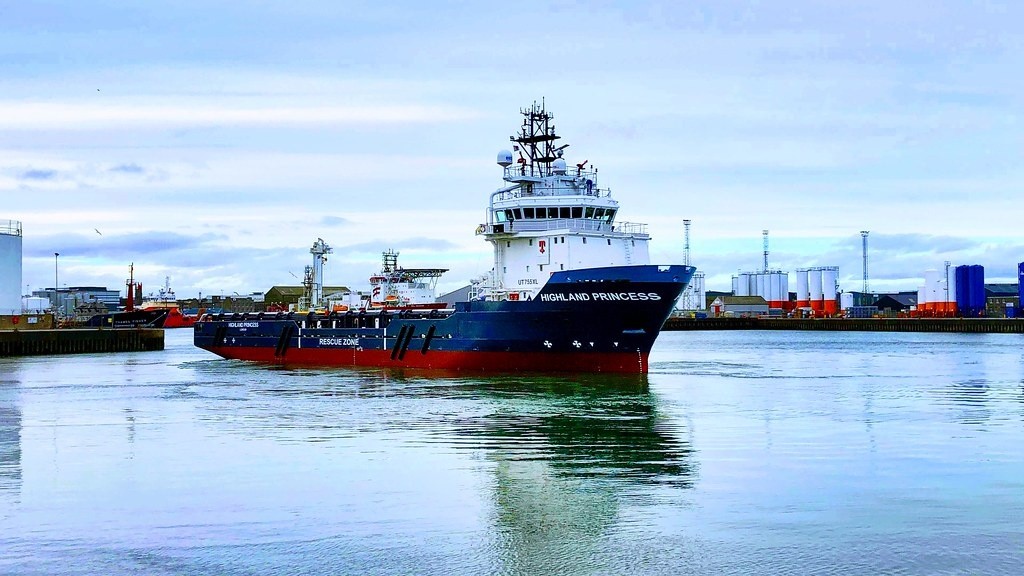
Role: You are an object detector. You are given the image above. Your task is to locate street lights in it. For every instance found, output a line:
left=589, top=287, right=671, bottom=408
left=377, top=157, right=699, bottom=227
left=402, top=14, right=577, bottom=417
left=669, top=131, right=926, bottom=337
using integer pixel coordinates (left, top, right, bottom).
left=55, top=252, right=59, bottom=305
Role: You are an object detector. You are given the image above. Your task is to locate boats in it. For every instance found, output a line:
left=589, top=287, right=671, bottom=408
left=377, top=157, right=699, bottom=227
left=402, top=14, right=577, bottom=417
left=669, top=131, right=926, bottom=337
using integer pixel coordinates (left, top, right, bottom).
left=194, top=96, right=697, bottom=372
left=64, top=262, right=210, bottom=329
